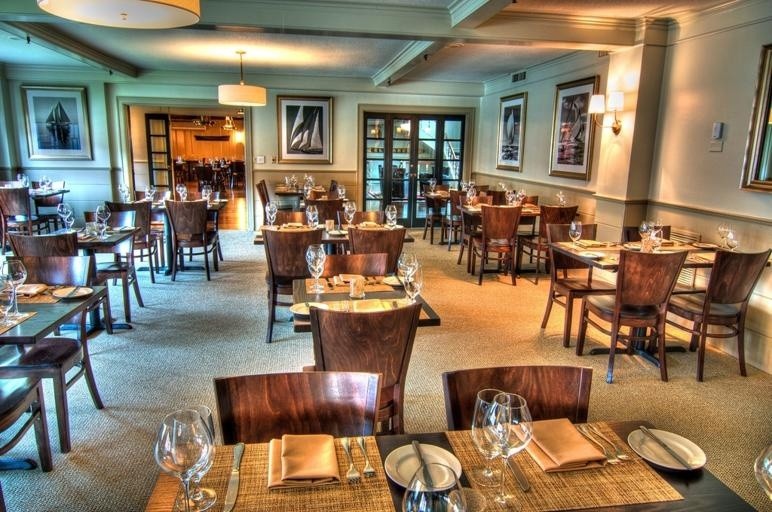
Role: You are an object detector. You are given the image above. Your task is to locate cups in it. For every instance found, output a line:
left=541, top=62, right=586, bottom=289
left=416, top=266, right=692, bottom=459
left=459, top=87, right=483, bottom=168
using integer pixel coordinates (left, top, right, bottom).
left=399, top=464, right=465, bottom=512
left=349, top=278, right=366, bottom=298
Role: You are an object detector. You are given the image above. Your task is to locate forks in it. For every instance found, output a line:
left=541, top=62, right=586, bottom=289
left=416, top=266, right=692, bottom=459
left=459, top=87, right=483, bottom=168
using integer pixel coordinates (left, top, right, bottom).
left=578, top=419, right=638, bottom=472
left=340, top=436, right=376, bottom=484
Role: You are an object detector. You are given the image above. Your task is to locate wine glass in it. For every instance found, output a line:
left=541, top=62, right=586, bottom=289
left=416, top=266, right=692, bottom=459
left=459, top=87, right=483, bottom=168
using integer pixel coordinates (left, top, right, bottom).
left=0, top=259, right=28, bottom=324
left=286, top=172, right=349, bottom=205
left=154, top=404, right=219, bottom=512
left=397, top=252, right=423, bottom=306
left=16, top=174, right=51, bottom=192
left=118, top=183, right=213, bottom=206
left=428, top=177, right=565, bottom=207
left=570, top=217, right=743, bottom=252
left=306, top=242, right=324, bottom=291
left=469, top=387, right=533, bottom=509
left=56, top=203, right=112, bottom=240
left=264, top=202, right=398, bottom=230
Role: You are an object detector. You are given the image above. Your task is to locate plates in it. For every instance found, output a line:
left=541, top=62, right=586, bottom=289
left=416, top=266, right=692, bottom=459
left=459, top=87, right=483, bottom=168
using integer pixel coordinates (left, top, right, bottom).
left=329, top=231, right=349, bottom=236
left=290, top=301, right=328, bottom=315
left=626, top=427, right=706, bottom=472
left=383, top=276, right=414, bottom=285
left=579, top=251, right=604, bottom=259
left=384, top=443, right=462, bottom=493
left=52, top=287, right=95, bottom=299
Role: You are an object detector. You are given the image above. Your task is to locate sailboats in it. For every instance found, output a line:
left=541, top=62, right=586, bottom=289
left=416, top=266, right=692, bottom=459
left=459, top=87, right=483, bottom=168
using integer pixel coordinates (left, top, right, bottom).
left=47, top=125, right=71, bottom=145
left=560, top=105, right=584, bottom=146
left=503, top=109, right=516, bottom=151
left=46, top=102, right=72, bottom=130
left=291, top=106, right=323, bottom=154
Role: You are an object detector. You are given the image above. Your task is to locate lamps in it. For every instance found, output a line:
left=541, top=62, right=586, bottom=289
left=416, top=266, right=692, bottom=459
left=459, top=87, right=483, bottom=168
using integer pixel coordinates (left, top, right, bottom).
left=217, top=51, right=268, bottom=107
left=586, top=91, right=626, bottom=136
left=35, top=1, right=202, bottom=32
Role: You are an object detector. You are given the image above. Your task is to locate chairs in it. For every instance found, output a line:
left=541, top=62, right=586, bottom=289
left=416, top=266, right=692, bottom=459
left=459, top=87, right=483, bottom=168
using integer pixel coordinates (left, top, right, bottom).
left=1, top=159, right=160, bottom=335
left=443, top=364, right=592, bottom=431
left=3, top=256, right=104, bottom=452
left=255, top=178, right=442, bottom=344
left=542, top=224, right=772, bottom=383
left=212, top=370, right=383, bottom=446
left=2, top=377, right=53, bottom=511
left=421, top=185, right=578, bottom=286
left=304, top=303, right=422, bottom=435
left=156, top=159, right=246, bottom=280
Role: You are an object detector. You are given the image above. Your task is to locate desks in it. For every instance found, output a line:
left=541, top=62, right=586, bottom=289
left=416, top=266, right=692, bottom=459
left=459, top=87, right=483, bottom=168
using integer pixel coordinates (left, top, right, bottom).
left=1, top=281, right=109, bottom=354
left=143, top=422, right=756, bottom=512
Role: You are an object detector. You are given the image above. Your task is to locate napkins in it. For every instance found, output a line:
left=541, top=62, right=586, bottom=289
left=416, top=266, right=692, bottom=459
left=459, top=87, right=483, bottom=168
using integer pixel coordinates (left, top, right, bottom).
left=15, top=283, right=46, bottom=299
left=267, top=434, right=340, bottom=490
left=513, top=418, right=607, bottom=473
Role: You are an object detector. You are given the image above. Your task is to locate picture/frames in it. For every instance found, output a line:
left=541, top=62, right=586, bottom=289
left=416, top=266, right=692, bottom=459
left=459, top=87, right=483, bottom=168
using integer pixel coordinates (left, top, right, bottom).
left=547, top=73, right=601, bottom=183
left=737, top=42, right=772, bottom=195
left=20, top=83, right=96, bottom=162
left=275, top=94, right=335, bottom=166
left=495, top=89, right=529, bottom=174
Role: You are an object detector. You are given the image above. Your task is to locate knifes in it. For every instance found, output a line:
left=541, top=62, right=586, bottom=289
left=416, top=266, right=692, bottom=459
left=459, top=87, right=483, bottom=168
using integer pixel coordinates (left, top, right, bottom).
left=639, top=424, right=696, bottom=475
left=221, top=441, right=248, bottom=511
left=409, top=439, right=435, bottom=489
